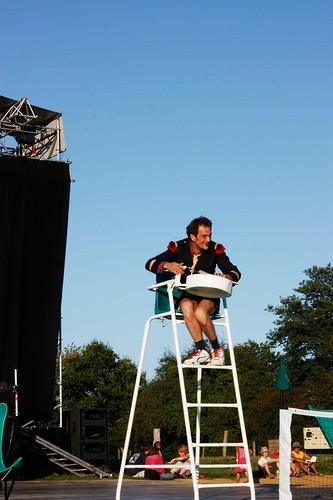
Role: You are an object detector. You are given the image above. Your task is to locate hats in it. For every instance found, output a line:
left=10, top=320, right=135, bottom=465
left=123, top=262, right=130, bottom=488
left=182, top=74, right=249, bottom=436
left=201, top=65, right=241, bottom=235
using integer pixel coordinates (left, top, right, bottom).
left=292, top=442, right=303, bottom=447
left=260, top=447, right=270, bottom=453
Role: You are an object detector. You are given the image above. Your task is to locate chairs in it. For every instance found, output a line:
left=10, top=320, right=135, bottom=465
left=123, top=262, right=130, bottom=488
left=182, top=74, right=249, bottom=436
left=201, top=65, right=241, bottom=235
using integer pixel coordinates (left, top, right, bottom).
left=155, top=273, right=220, bottom=320
left=0, top=403, right=23, bottom=500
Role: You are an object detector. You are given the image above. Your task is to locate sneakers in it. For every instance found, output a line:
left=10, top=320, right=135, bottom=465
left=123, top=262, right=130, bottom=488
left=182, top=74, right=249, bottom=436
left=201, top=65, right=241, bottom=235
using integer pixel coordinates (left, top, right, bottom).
left=183, top=349, right=211, bottom=366
left=210, top=347, right=225, bottom=366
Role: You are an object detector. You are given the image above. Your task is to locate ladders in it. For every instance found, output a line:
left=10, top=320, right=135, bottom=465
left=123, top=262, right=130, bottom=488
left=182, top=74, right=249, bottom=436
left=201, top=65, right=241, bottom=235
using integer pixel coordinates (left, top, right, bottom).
left=22, top=421, right=113, bottom=479
left=115, top=266, right=256, bottom=500
left=54, top=326, right=63, bottom=426
left=0, top=97, right=75, bottom=182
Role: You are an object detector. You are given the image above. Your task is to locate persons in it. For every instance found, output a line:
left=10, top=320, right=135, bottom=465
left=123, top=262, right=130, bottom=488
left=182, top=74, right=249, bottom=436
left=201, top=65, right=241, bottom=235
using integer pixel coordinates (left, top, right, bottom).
left=145, top=216, right=241, bottom=367
left=170, top=445, right=192, bottom=479
left=235, top=433, right=252, bottom=483
left=257, top=442, right=320, bottom=479
left=124, top=441, right=174, bottom=480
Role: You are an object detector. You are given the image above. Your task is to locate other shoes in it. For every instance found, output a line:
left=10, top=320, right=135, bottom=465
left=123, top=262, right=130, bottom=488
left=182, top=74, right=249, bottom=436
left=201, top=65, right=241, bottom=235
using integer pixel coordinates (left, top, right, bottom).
left=290, top=473, right=299, bottom=477
left=316, top=473, right=321, bottom=476
left=269, top=475, right=275, bottom=478
left=309, top=473, right=315, bottom=476
